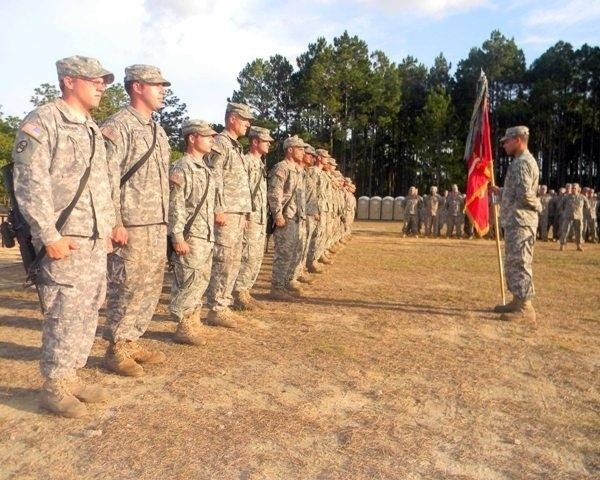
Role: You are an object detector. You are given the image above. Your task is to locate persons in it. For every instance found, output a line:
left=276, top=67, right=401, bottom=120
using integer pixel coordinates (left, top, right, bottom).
left=231, top=124, right=274, bottom=315
left=398, top=179, right=600, bottom=253
left=203, top=102, right=258, bottom=332
left=9, top=53, right=118, bottom=422
left=168, top=118, right=218, bottom=351
left=97, top=63, right=174, bottom=380
left=266, top=136, right=360, bottom=303
left=488, top=122, right=541, bottom=325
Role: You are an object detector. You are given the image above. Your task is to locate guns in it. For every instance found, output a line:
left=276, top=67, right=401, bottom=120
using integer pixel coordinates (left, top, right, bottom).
left=166, top=235, right=183, bottom=293
left=266, top=175, right=276, bottom=253
left=0, top=161, right=46, bottom=314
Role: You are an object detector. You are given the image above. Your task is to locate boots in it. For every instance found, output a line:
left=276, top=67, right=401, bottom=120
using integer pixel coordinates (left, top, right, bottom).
left=494, top=297, right=515, bottom=313
left=59, top=372, right=108, bottom=405
left=173, top=316, right=206, bottom=346
left=270, top=232, right=356, bottom=301
left=100, top=338, right=143, bottom=377
left=499, top=299, right=537, bottom=323
left=560, top=243, right=566, bottom=251
left=123, top=339, right=167, bottom=365
left=576, top=243, right=584, bottom=251
left=188, top=312, right=216, bottom=337
left=38, top=376, right=88, bottom=419
left=206, top=304, right=251, bottom=330
left=233, top=291, right=269, bottom=311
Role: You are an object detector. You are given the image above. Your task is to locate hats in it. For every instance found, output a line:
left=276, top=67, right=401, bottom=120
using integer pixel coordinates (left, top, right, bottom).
left=180, top=117, right=219, bottom=138
left=226, top=101, right=254, bottom=121
left=249, top=126, right=276, bottom=143
left=283, top=136, right=357, bottom=191
left=499, top=126, right=531, bottom=143
left=55, top=56, right=114, bottom=86
left=122, top=64, right=172, bottom=87
left=406, top=182, right=599, bottom=196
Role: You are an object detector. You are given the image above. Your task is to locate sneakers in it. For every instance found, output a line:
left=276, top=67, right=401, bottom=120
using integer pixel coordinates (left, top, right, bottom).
left=400, top=229, right=600, bottom=243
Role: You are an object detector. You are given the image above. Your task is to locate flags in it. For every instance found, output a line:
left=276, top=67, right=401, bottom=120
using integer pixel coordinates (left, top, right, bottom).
left=459, top=66, right=499, bottom=241
